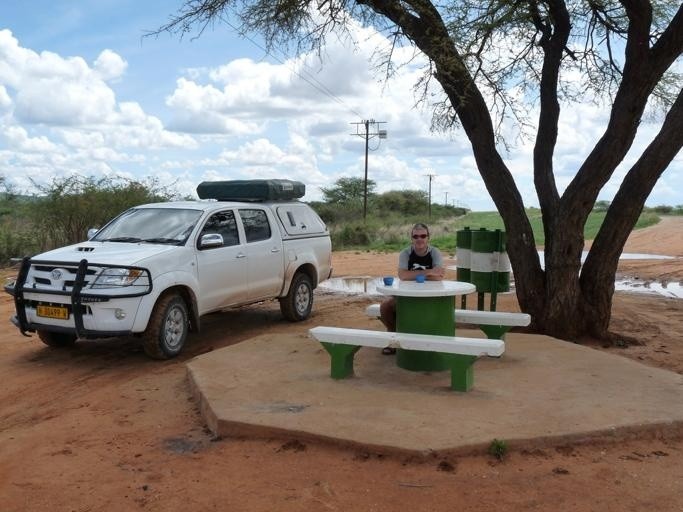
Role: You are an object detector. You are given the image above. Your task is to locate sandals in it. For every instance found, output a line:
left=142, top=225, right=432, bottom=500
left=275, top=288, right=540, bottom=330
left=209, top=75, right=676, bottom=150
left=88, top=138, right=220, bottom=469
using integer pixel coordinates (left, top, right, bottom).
left=381, top=347, right=396, bottom=355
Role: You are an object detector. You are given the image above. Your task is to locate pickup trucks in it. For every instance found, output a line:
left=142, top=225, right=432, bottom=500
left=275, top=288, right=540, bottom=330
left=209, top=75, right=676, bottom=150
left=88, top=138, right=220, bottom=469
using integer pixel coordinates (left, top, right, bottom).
left=3, top=178, right=335, bottom=361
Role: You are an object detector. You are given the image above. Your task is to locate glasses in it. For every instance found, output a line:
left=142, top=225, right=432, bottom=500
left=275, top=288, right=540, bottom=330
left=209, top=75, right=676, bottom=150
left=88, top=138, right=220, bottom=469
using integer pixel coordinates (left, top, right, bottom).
left=411, top=230, right=428, bottom=239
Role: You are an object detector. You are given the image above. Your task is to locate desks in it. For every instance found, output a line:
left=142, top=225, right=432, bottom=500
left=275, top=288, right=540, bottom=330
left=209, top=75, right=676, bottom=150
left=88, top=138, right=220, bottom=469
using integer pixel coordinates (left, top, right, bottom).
left=377, top=279, right=476, bottom=372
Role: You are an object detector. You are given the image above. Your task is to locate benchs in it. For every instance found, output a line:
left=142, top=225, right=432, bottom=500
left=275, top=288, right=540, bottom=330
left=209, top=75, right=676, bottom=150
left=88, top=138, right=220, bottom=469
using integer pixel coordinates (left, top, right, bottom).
left=309, top=304, right=531, bottom=392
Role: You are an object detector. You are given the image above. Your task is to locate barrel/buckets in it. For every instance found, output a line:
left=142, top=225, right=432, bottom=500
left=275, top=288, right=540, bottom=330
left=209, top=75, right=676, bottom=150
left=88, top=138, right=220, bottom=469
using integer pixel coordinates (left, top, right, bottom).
left=456, top=228, right=480, bottom=283
left=490, top=229, right=510, bottom=293
left=470, top=227, right=495, bottom=293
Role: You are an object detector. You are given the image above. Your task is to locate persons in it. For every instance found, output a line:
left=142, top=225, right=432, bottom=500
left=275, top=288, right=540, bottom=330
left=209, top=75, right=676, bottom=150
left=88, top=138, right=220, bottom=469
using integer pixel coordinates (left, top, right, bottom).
left=378, top=221, right=445, bottom=354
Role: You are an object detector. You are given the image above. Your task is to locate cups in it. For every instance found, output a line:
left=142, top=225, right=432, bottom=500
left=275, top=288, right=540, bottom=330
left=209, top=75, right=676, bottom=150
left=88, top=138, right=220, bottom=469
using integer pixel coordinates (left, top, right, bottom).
left=416, top=275, right=424, bottom=283
left=384, top=277, right=393, bottom=285
